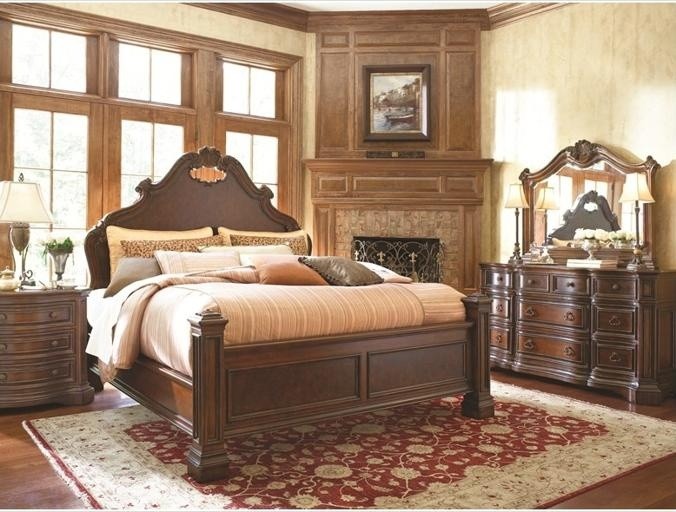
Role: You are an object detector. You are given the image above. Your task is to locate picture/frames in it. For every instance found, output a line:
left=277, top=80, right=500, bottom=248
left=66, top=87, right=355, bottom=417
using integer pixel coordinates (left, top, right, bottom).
left=358, top=60, right=434, bottom=145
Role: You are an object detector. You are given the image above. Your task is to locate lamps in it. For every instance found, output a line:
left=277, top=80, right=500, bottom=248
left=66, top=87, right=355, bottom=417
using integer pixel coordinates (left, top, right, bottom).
left=0, top=171, right=58, bottom=291
left=501, top=183, right=531, bottom=263
left=618, top=171, right=658, bottom=274
left=535, top=187, right=562, bottom=245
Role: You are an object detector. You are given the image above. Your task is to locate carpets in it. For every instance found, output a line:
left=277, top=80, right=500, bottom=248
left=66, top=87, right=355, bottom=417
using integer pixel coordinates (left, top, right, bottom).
left=21, top=375, right=676, bottom=509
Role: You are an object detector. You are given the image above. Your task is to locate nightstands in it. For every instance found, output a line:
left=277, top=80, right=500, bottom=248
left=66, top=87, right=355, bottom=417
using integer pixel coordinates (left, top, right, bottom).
left=0, top=290, right=94, bottom=413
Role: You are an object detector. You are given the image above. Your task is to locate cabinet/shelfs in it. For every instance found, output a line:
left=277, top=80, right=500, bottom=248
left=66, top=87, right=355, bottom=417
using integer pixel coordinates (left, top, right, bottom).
left=471, top=263, right=676, bottom=404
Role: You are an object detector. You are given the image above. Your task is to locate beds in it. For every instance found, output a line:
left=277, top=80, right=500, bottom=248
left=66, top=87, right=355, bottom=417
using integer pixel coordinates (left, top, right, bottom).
left=81, top=147, right=499, bottom=487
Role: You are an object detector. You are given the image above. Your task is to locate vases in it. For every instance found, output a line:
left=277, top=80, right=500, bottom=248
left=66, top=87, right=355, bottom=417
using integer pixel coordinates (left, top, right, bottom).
left=50, top=254, right=70, bottom=285
left=581, top=240, right=601, bottom=261
left=614, top=241, right=630, bottom=250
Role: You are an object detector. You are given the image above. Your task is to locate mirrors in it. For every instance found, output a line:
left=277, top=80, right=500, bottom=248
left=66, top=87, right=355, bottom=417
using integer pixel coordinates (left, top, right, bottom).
left=520, top=140, right=658, bottom=262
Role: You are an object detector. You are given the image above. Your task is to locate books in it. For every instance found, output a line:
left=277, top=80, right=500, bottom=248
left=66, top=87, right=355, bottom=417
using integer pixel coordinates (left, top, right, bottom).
left=564, top=258, right=619, bottom=269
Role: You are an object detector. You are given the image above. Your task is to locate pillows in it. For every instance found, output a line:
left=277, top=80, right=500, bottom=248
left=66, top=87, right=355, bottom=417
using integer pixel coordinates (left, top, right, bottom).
left=104, top=223, right=215, bottom=283
left=298, top=252, right=385, bottom=287
left=119, top=234, right=226, bottom=259
left=153, top=249, right=244, bottom=276
left=353, top=259, right=415, bottom=284
left=99, top=255, right=162, bottom=299
left=255, top=256, right=329, bottom=287
left=231, top=233, right=308, bottom=258
left=216, top=224, right=311, bottom=258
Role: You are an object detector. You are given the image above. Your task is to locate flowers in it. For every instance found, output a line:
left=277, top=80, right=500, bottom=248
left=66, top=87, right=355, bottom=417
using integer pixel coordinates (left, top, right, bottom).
left=609, top=230, right=640, bottom=242
left=572, top=226, right=609, bottom=242
left=34, top=235, right=78, bottom=262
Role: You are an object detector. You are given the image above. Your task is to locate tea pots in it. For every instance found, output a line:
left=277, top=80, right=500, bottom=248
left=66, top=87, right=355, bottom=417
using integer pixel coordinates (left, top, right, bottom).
left=0, top=265, right=21, bottom=291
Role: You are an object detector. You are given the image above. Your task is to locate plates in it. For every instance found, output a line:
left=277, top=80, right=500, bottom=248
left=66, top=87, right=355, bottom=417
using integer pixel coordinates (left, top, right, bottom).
left=58, top=285, right=78, bottom=290
